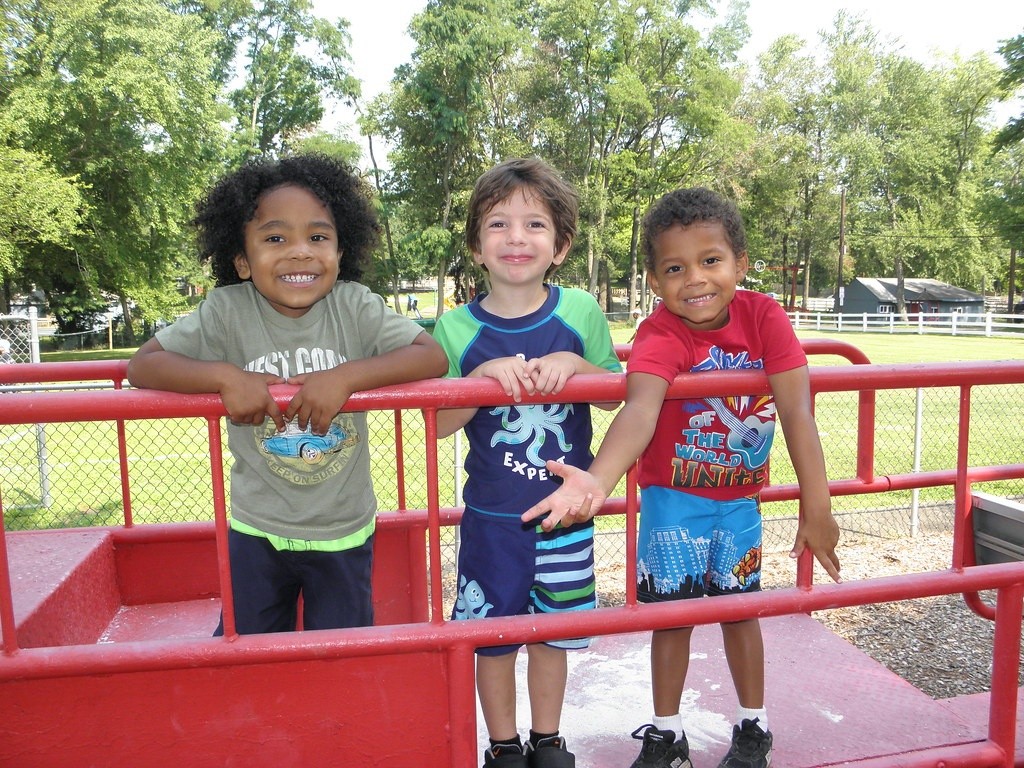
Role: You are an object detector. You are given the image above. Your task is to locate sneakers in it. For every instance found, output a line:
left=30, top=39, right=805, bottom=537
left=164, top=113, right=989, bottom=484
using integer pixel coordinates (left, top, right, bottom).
left=714, top=720, right=773, bottom=768
left=625, top=723, right=692, bottom=768
left=482, top=735, right=527, bottom=768
left=522, top=738, right=575, bottom=768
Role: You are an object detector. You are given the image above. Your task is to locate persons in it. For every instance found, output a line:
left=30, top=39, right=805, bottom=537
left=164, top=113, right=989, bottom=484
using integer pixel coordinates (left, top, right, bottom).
left=127, top=150, right=451, bottom=638
left=525, top=187, right=844, bottom=767
left=418, top=159, right=626, bottom=767
left=406, top=277, right=486, bottom=317
